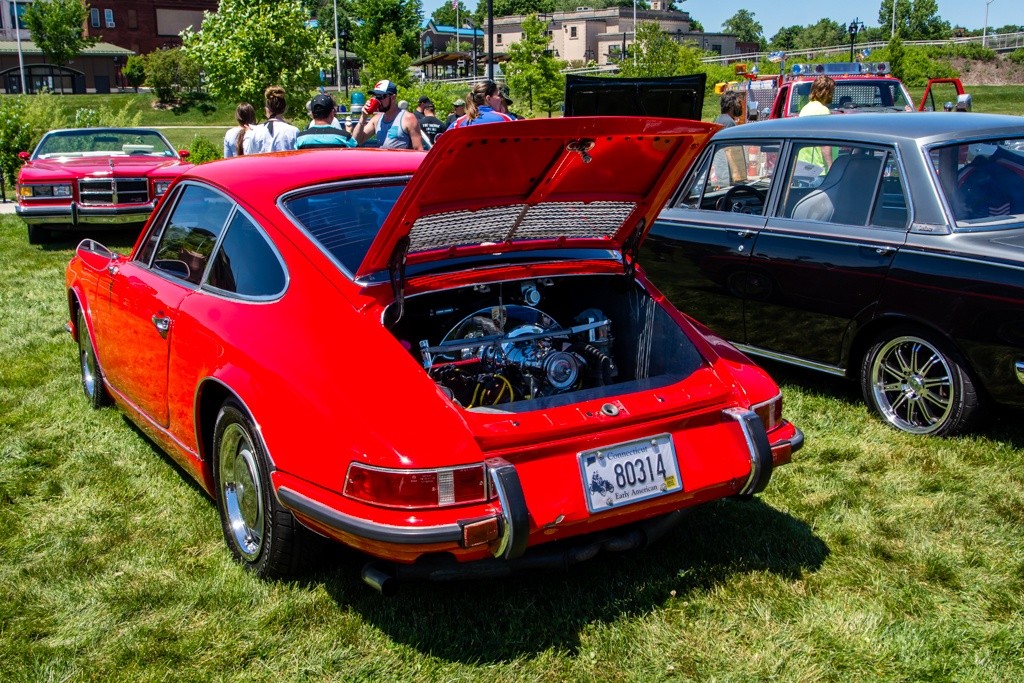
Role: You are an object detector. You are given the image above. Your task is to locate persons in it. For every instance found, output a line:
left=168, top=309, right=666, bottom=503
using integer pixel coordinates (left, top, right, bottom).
left=296, top=94, right=360, bottom=150
left=398, top=96, right=466, bottom=148
left=712, top=90, right=747, bottom=186
left=224, top=101, right=258, bottom=158
left=798, top=75, right=835, bottom=186
left=306, top=100, right=342, bottom=130
left=496, top=84, right=525, bottom=121
left=447, top=80, right=518, bottom=129
left=352, top=80, right=424, bottom=152
left=247, top=86, right=300, bottom=155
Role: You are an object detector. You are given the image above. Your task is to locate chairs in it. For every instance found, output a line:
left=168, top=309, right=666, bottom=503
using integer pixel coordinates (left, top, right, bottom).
left=791, top=153, right=883, bottom=229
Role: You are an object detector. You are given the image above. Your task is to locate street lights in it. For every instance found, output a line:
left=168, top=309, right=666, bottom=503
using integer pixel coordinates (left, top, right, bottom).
left=849, top=21, right=859, bottom=62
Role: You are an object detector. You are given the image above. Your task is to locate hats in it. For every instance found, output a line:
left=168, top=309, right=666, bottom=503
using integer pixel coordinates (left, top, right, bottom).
left=497, top=84, right=513, bottom=105
left=418, top=96, right=434, bottom=108
left=367, top=80, right=397, bottom=97
left=311, top=94, right=335, bottom=115
left=451, top=99, right=465, bottom=106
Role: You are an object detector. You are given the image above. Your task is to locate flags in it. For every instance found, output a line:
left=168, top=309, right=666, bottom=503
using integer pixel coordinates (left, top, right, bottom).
left=452, top=0, right=458, bottom=10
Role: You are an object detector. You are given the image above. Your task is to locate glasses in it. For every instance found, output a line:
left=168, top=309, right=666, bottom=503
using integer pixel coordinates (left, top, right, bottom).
left=372, top=93, right=388, bottom=99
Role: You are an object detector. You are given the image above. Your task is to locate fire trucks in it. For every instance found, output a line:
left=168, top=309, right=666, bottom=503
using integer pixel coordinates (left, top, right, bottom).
left=714, top=49, right=966, bottom=184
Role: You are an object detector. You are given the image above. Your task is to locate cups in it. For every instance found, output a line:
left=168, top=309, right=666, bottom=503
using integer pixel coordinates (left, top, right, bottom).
left=364, top=97, right=381, bottom=114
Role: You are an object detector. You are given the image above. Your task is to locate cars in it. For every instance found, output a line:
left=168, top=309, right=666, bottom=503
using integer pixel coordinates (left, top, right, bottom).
left=634, top=111, right=1024, bottom=436
left=63, top=114, right=805, bottom=583
left=16, top=127, right=196, bottom=244
